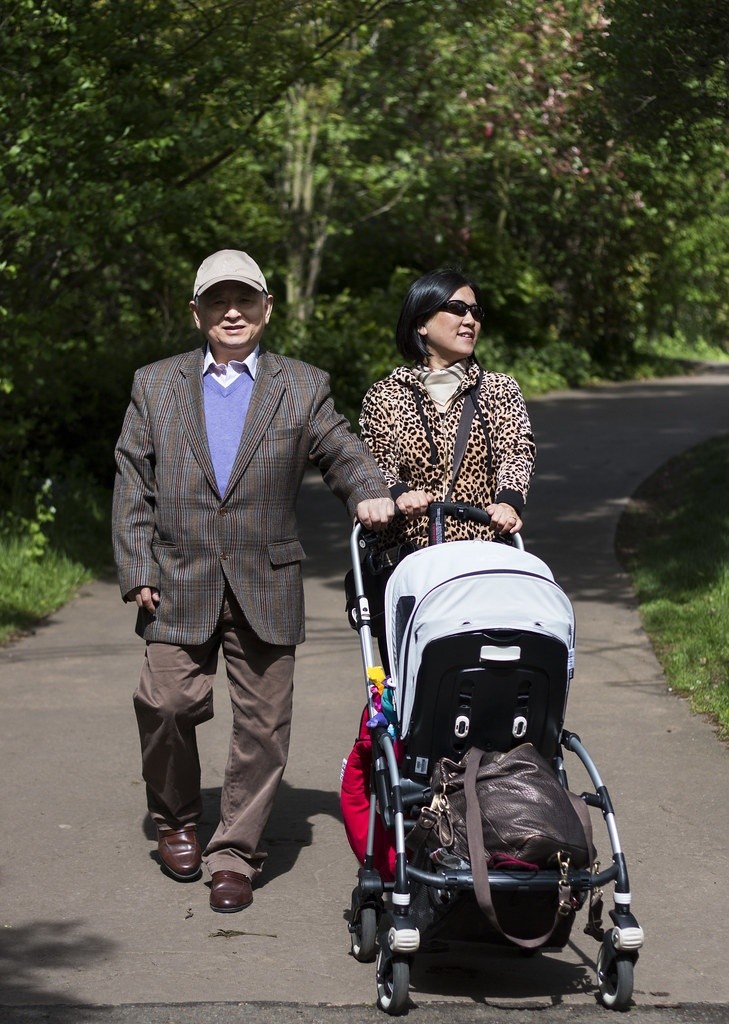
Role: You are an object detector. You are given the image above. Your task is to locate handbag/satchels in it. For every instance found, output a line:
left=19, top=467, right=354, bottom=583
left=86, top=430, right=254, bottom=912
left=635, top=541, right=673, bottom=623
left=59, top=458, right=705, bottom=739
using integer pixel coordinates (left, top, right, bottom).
left=404, top=742, right=598, bottom=949
left=343, top=543, right=414, bottom=636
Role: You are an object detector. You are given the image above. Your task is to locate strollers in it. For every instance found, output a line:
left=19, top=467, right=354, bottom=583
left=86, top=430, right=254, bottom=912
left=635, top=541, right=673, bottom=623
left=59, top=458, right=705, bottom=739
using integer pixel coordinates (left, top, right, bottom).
left=344, top=501, right=646, bottom=1015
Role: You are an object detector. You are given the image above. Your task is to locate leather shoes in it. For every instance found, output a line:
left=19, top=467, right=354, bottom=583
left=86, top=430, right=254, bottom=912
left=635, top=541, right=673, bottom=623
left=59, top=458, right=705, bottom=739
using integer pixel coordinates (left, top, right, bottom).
left=156, top=825, right=202, bottom=882
left=209, top=871, right=254, bottom=912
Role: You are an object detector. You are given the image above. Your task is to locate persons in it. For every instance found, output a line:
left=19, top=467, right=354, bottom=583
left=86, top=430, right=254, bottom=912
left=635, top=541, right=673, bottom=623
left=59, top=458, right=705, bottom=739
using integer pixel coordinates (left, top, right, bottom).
left=109, top=246, right=392, bottom=914
left=356, top=268, right=537, bottom=609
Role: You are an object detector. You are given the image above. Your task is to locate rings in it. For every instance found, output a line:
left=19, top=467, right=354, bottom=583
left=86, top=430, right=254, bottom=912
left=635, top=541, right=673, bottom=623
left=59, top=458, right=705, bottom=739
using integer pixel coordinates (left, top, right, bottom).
left=509, top=516, right=519, bottom=521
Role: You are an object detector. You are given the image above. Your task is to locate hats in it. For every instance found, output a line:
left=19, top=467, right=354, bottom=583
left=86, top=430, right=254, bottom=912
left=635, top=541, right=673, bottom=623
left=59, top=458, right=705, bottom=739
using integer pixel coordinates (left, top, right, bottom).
left=192, top=249, right=269, bottom=303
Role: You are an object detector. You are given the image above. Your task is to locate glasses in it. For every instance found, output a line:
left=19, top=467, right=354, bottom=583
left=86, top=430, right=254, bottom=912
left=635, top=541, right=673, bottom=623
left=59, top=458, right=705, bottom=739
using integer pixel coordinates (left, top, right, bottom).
left=437, top=300, right=485, bottom=322
